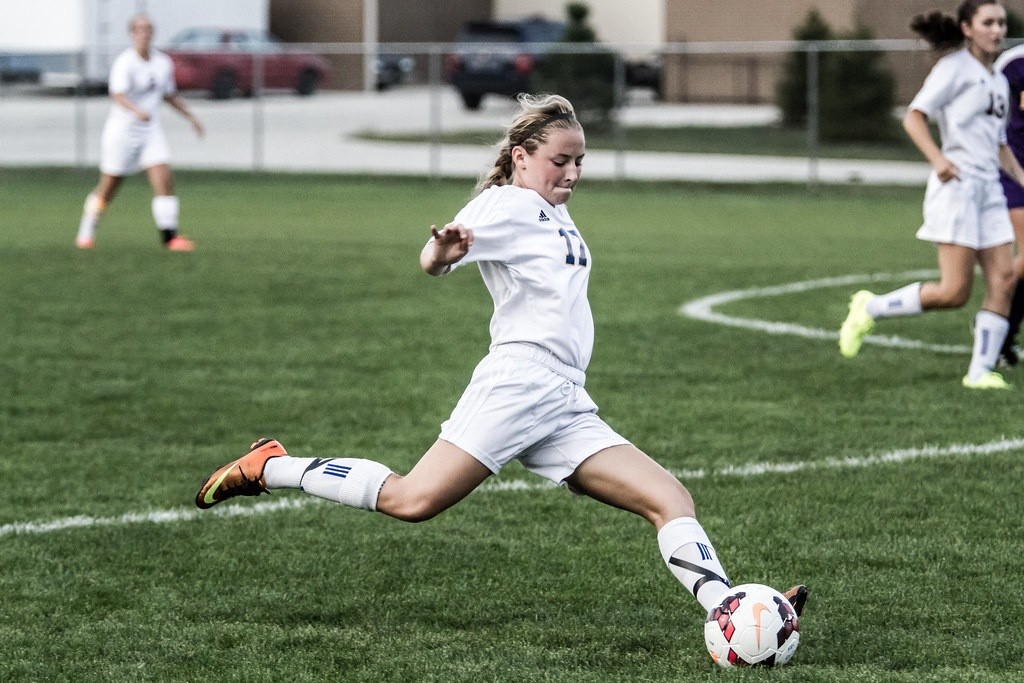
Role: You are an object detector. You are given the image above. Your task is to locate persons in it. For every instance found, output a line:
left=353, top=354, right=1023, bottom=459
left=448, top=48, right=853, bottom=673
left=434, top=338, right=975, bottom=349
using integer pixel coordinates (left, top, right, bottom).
left=971, top=43, right=1023, bottom=370
left=76, top=9, right=197, bottom=252
left=196, top=92, right=808, bottom=670
left=835, top=0, right=1023, bottom=394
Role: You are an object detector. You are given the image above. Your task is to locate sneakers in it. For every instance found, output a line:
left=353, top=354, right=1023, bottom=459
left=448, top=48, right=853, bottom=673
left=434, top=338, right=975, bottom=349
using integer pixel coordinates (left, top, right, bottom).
left=836, top=288, right=880, bottom=359
left=194, top=432, right=287, bottom=510
left=76, top=231, right=99, bottom=250
left=774, top=585, right=812, bottom=627
left=962, top=371, right=1017, bottom=395
left=160, top=234, right=199, bottom=253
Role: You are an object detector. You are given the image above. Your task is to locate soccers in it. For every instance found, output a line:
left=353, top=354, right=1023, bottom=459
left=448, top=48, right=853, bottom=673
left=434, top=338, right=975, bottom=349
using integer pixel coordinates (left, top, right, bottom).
left=704, top=583, right=800, bottom=671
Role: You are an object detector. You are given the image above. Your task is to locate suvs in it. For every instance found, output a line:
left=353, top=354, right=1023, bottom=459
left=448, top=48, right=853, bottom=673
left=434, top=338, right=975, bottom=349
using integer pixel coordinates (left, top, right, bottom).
left=444, top=11, right=629, bottom=114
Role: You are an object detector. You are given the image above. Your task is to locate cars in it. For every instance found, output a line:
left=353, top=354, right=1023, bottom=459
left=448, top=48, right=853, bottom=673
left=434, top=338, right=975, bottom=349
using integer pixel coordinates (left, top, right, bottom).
left=161, top=24, right=329, bottom=101
left=375, top=54, right=418, bottom=94
left=624, top=46, right=665, bottom=94
